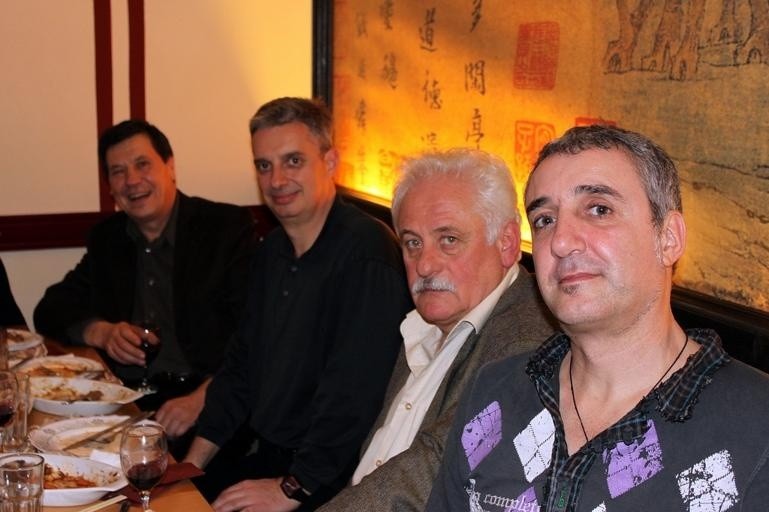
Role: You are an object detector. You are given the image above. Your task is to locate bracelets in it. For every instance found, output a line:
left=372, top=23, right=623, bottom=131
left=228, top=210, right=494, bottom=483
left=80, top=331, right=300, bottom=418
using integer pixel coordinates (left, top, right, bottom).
left=280, top=476, right=309, bottom=502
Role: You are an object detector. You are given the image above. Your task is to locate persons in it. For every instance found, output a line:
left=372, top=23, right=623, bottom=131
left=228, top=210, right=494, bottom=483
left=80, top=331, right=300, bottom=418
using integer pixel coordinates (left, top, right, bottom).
left=33, top=120, right=239, bottom=462
left=182, top=99, right=418, bottom=511
left=425, top=124, right=767, bottom=511
left=314, top=146, right=561, bottom=511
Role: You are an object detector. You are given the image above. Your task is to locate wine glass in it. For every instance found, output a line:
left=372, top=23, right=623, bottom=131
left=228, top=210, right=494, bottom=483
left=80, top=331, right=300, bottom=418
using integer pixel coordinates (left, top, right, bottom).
left=118, top=419, right=170, bottom=511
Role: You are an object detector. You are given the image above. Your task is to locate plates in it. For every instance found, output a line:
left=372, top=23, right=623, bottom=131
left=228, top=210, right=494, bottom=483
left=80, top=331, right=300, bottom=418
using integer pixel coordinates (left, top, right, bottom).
left=6, top=326, right=45, bottom=351
left=17, top=353, right=146, bottom=417
left=0, top=414, right=165, bottom=508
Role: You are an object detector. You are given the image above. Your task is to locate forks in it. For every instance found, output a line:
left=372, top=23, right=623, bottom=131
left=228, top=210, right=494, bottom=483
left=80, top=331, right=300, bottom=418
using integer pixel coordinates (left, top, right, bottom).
left=88, top=409, right=157, bottom=447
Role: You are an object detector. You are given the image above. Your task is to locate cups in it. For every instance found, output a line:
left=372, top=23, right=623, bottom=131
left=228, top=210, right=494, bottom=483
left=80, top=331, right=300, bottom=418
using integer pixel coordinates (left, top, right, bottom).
left=0, top=371, right=32, bottom=454
